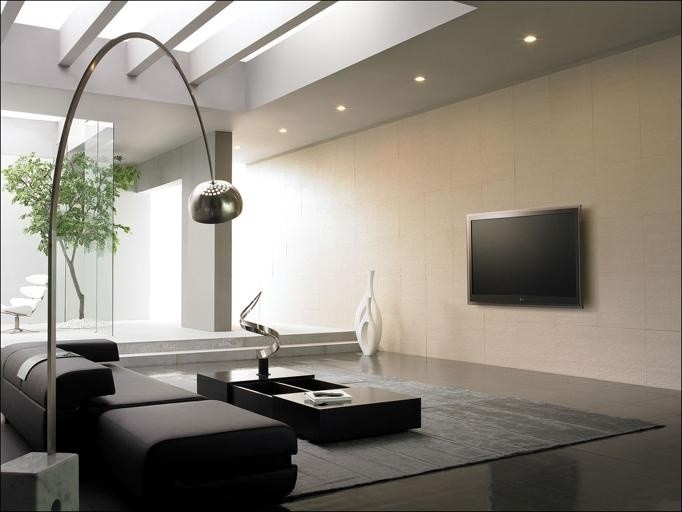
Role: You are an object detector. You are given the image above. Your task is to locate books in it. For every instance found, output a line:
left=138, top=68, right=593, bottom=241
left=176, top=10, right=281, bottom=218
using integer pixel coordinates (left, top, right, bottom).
left=305, top=389, right=352, bottom=403
left=304, top=399, right=351, bottom=406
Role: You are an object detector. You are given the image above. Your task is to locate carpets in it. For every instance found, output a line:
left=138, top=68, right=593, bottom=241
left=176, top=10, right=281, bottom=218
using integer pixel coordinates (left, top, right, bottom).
left=123, top=355, right=667, bottom=499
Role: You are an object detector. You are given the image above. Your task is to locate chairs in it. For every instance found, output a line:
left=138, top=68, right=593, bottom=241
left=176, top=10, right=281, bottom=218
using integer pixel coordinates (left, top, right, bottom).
left=1, top=273, right=48, bottom=334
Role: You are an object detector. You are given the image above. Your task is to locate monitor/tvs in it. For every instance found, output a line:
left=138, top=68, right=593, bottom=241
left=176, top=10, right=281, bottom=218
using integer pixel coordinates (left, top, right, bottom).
left=466, top=203, right=583, bottom=309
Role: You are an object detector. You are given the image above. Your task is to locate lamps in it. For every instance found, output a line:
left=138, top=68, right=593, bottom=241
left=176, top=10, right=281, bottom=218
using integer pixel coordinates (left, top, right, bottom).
left=0, top=31, right=243, bottom=512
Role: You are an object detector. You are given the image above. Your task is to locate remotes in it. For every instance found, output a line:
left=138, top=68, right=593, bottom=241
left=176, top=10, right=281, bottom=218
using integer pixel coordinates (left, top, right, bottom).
left=312, top=392, right=344, bottom=397
